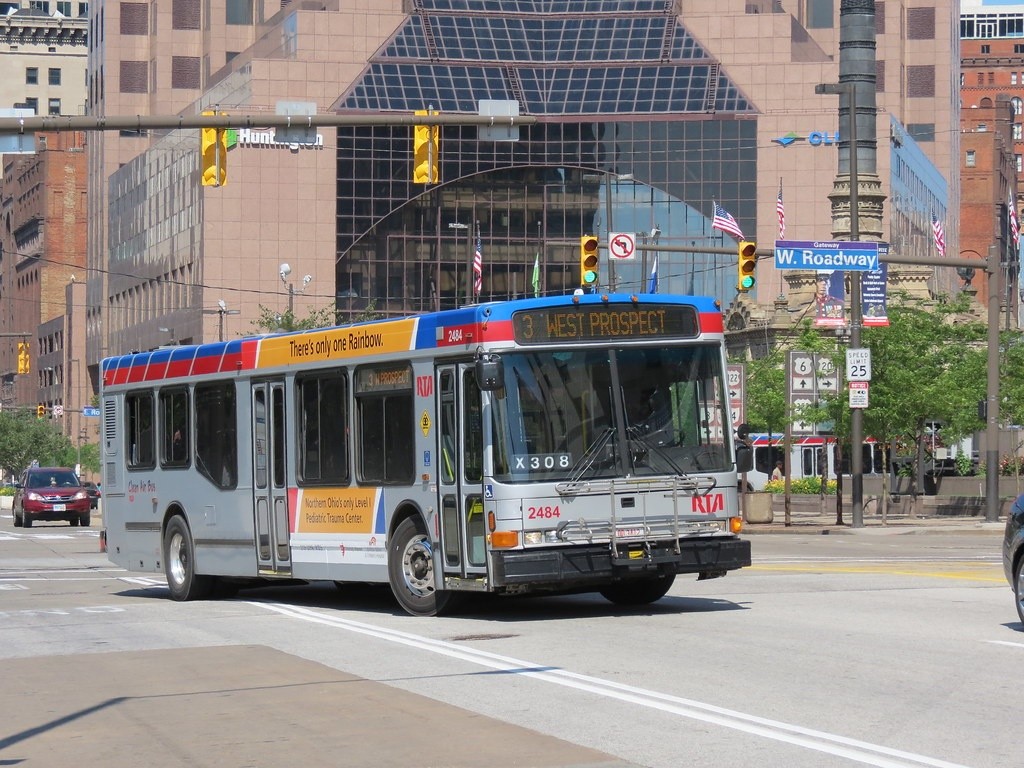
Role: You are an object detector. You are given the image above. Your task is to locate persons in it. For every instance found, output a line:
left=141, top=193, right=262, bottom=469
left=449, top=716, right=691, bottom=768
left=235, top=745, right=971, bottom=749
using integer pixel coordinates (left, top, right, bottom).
left=772, top=460, right=784, bottom=481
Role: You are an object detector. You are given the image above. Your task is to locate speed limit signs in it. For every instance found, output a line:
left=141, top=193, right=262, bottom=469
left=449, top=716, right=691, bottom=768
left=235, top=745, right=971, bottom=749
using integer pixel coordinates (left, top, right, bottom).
left=845, top=349, right=871, bottom=381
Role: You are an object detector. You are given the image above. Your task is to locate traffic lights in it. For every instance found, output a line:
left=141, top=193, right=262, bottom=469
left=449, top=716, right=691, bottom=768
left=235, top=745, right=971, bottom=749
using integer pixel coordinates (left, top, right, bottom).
left=37, top=406, right=44, bottom=420
left=581, top=236, right=599, bottom=285
left=738, top=243, right=756, bottom=290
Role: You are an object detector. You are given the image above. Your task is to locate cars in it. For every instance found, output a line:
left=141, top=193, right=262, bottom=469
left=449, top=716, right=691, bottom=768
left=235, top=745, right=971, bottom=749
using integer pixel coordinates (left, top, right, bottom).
left=1002, top=494, right=1024, bottom=625
left=81, top=481, right=101, bottom=508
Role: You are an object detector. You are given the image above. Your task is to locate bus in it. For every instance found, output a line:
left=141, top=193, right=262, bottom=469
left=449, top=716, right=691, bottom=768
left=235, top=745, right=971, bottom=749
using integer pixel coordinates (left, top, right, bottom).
left=98, top=289, right=754, bottom=613
left=734, top=432, right=944, bottom=493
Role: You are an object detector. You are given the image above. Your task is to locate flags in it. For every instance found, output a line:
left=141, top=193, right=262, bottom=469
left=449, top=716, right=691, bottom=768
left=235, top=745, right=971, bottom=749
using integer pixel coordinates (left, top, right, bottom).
left=776, top=177, right=786, bottom=240
left=712, top=200, right=745, bottom=242
left=648, top=243, right=658, bottom=294
left=532, top=251, right=540, bottom=299
left=1009, top=186, right=1018, bottom=244
left=473, top=225, right=482, bottom=296
left=932, top=206, right=946, bottom=257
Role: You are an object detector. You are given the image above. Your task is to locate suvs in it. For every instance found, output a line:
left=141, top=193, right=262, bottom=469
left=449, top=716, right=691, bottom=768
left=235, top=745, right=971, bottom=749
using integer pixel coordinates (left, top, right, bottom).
left=11, top=466, right=90, bottom=528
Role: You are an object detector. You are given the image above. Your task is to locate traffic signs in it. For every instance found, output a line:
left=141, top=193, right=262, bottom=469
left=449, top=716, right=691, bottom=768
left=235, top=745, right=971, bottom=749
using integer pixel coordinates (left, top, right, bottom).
left=786, top=351, right=837, bottom=434
left=698, top=364, right=747, bottom=445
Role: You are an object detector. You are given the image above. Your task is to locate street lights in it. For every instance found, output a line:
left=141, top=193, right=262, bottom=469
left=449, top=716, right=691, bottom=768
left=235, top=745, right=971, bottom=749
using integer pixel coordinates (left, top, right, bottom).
left=71, top=359, right=81, bottom=484
left=199, top=309, right=240, bottom=340
left=638, top=228, right=661, bottom=294
left=583, top=174, right=633, bottom=294
left=44, top=368, right=55, bottom=431
left=159, top=327, right=174, bottom=343
left=448, top=222, right=472, bottom=304
left=280, top=264, right=312, bottom=332
left=816, top=83, right=864, bottom=527
